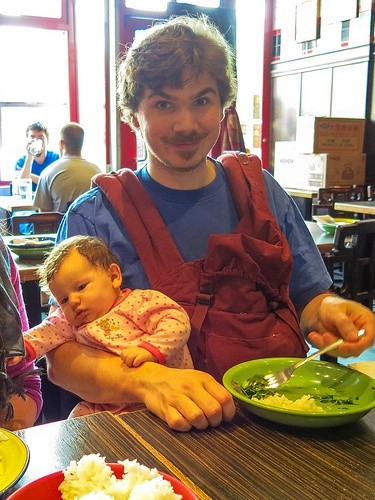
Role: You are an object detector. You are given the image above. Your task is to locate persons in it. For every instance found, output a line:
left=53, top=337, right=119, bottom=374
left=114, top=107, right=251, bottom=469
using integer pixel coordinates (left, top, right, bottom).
left=10, top=234, right=194, bottom=421
left=33, top=124, right=101, bottom=213
left=0, top=231, right=44, bottom=432
left=46, top=18, right=375, bottom=432
left=14, top=122, right=60, bottom=234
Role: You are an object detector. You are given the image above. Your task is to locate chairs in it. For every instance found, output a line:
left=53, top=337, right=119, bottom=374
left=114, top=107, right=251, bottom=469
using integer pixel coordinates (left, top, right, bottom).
left=12, top=211, right=66, bottom=235
left=322, top=220, right=375, bottom=303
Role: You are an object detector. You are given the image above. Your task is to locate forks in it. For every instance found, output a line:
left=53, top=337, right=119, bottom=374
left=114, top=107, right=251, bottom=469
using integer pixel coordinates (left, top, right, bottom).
left=263, top=329, right=365, bottom=389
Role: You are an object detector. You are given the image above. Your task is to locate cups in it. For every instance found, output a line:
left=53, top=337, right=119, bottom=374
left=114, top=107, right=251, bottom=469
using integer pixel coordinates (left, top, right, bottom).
left=19, top=177, right=32, bottom=201
left=28, top=138, right=43, bottom=156
left=11, top=179, right=20, bottom=202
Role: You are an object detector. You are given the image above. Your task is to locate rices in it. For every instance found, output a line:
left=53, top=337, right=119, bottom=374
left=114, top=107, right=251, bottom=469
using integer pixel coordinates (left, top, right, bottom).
left=58, top=453, right=184, bottom=500
left=249, top=393, right=325, bottom=411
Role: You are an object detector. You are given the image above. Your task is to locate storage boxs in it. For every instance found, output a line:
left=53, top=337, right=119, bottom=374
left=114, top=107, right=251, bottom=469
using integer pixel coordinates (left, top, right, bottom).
left=274, top=116, right=366, bottom=190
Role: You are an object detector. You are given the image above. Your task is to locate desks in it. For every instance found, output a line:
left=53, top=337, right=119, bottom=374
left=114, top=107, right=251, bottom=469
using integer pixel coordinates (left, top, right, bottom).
left=0, top=360, right=375, bottom=500
left=281, top=186, right=375, bottom=266
left=1, top=232, right=61, bottom=287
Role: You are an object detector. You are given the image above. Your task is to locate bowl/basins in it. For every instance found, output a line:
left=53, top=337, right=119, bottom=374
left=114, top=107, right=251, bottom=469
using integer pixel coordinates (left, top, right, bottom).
left=7, top=236, right=56, bottom=259
left=222, top=357, right=375, bottom=428
left=317, top=218, right=359, bottom=233
